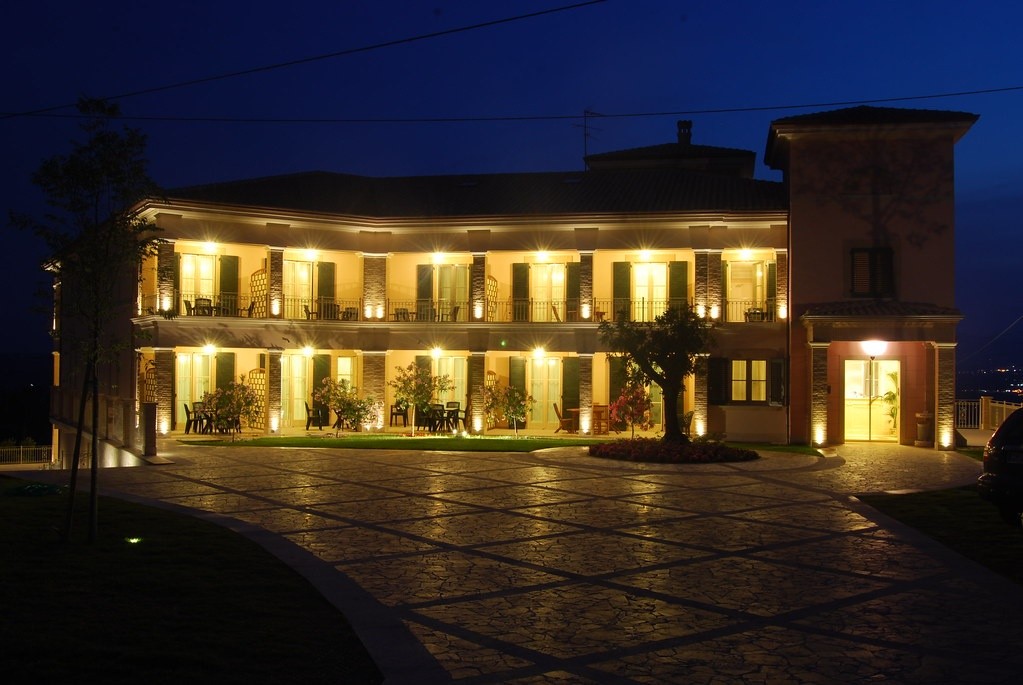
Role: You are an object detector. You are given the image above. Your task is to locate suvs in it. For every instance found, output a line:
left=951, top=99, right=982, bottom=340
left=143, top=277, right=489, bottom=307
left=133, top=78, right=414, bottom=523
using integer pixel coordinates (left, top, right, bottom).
left=979, top=406, right=1023, bottom=524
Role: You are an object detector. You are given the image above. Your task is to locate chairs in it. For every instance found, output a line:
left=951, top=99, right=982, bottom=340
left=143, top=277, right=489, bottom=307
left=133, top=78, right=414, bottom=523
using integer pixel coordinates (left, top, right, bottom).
left=390, top=394, right=470, bottom=432
left=748, top=307, right=763, bottom=323
left=664, top=410, right=695, bottom=437
left=184, top=402, right=242, bottom=436
left=552, top=403, right=622, bottom=437
left=304, top=303, right=359, bottom=321
left=304, top=401, right=358, bottom=433
left=183, top=297, right=257, bottom=318
left=394, top=305, right=461, bottom=323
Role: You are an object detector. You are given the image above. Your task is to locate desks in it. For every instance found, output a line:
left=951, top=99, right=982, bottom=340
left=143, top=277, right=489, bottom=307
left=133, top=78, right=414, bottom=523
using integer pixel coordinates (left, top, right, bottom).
left=744, top=311, right=765, bottom=323
left=191, top=305, right=232, bottom=317
left=430, top=407, right=460, bottom=432
left=566, top=408, right=604, bottom=435
left=190, top=409, right=217, bottom=434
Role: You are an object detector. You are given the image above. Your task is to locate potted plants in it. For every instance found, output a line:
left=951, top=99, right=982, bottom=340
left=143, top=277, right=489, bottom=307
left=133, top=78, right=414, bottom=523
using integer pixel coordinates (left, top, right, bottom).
left=868, top=371, right=899, bottom=436
